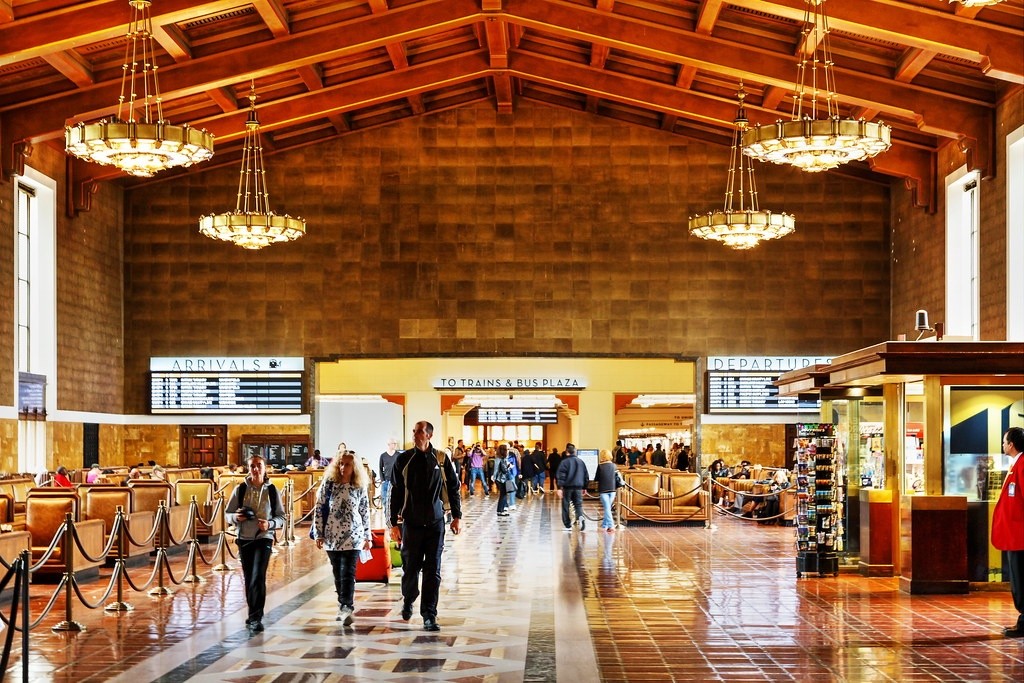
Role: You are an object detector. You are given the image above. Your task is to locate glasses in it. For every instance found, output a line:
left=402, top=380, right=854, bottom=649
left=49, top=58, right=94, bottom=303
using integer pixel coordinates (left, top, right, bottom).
left=343, top=449, right=354, bottom=454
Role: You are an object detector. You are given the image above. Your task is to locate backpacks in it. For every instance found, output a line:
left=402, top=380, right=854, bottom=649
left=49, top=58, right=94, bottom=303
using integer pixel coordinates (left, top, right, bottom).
left=516, top=478, right=528, bottom=499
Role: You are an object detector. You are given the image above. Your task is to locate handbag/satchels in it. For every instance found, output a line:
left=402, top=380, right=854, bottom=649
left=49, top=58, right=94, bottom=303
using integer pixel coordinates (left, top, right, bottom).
left=616, top=448, right=626, bottom=465
left=615, top=470, right=625, bottom=489
left=309, top=481, right=334, bottom=540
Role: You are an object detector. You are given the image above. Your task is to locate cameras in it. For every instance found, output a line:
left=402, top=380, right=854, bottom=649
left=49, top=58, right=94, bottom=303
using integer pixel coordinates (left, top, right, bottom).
left=240, top=507, right=255, bottom=519
left=475, top=445, right=480, bottom=448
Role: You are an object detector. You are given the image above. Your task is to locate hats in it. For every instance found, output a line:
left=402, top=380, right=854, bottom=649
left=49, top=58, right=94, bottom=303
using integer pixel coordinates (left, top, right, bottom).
left=656, top=443, right=662, bottom=448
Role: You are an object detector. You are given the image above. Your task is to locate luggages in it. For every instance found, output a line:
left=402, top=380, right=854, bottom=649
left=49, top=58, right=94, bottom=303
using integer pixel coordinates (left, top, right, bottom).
left=354, top=529, right=389, bottom=586
left=390, top=540, right=404, bottom=569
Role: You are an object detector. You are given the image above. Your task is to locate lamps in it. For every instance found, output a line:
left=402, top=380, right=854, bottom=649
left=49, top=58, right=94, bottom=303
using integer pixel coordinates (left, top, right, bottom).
left=740, top=0, right=893, bottom=173
left=686, top=78, right=796, bottom=249
left=198, top=78, right=308, bottom=250
left=62, top=0, right=215, bottom=178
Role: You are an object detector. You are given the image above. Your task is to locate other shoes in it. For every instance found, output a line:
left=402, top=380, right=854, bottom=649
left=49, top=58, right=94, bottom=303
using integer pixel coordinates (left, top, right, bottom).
left=251, top=621, right=263, bottom=631
left=562, top=526, right=572, bottom=531
left=470, top=492, right=474, bottom=496
left=742, top=511, right=752, bottom=518
left=336, top=606, right=342, bottom=621
left=342, top=610, right=353, bottom=627
left=506, top=480, right=518, bottom=492
left=423, top=618, right=440, bottom=631
left=484, top=493, right=490, bottom=497
left=579, top=516, right=585, bottom=531
left=531, top=486, right=545, bottom=493
left=497, top=504, right=516, bottom=516
left=607, top=528, right=614, bottom=532
left=246, top=618, right=250, bottom=624
left=402, top=600, right=414, bottom=621
left=1003, top=625, right=1022, bottom=637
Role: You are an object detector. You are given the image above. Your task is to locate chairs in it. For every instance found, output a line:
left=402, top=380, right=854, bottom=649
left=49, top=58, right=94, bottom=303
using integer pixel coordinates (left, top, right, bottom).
left=0, top=465, right=376, bottom=605
left=614, top=463, right=797, bottom=529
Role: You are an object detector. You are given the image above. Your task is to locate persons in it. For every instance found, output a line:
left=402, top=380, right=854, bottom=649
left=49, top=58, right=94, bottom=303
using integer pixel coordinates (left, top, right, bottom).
left=991, top=428, right=1023, bottom=635
left=316, top=449, right=373, bottom=627
left=225, top=453, right=286, bottom=632
left=48, top=439, right=796, bottom=532
left=389, top=421, right=463, bottom=632
